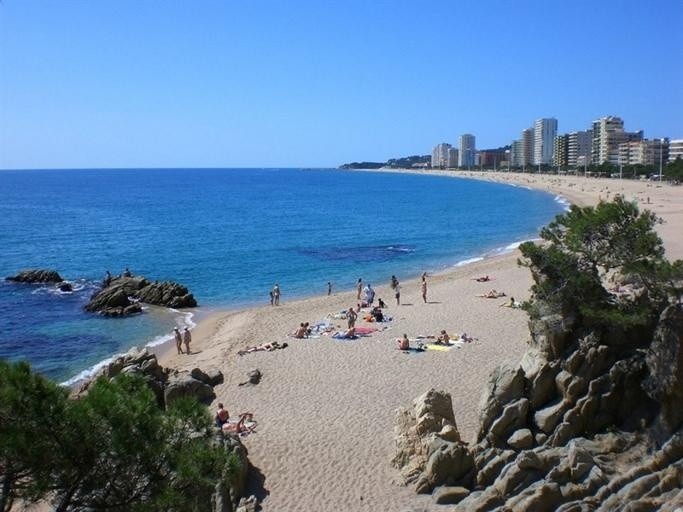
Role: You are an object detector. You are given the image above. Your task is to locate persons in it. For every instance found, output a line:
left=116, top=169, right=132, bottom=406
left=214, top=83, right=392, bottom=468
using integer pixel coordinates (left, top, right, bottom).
left=105, top=270, right=110, bottom=287
left=437, top=330, right=449, bottom=344
left=215, top=403, right=248, bottom=433
left=244, top=341, right=277, bottom=352
left=295, top=322, right=309, bottom=338
left=399, top=333, right=409, bottom=350
left=391, top=275, right=400, bottom=305
left=332, top=278, right=386, bottom=338
left=327, top=282, right=332, bottom=296
left=269, top=284, right=281, bottom=305
left=420, top=272, right=427, bottom=303
left=475, top=289, right=514, bottom=307
left=183, top=328, right=191, bottom=355
left=174, top=326, right=183, bottom=355
left=123, top=268, right=130, bottom=277
left=470, top=276, right=490, bottom=282
